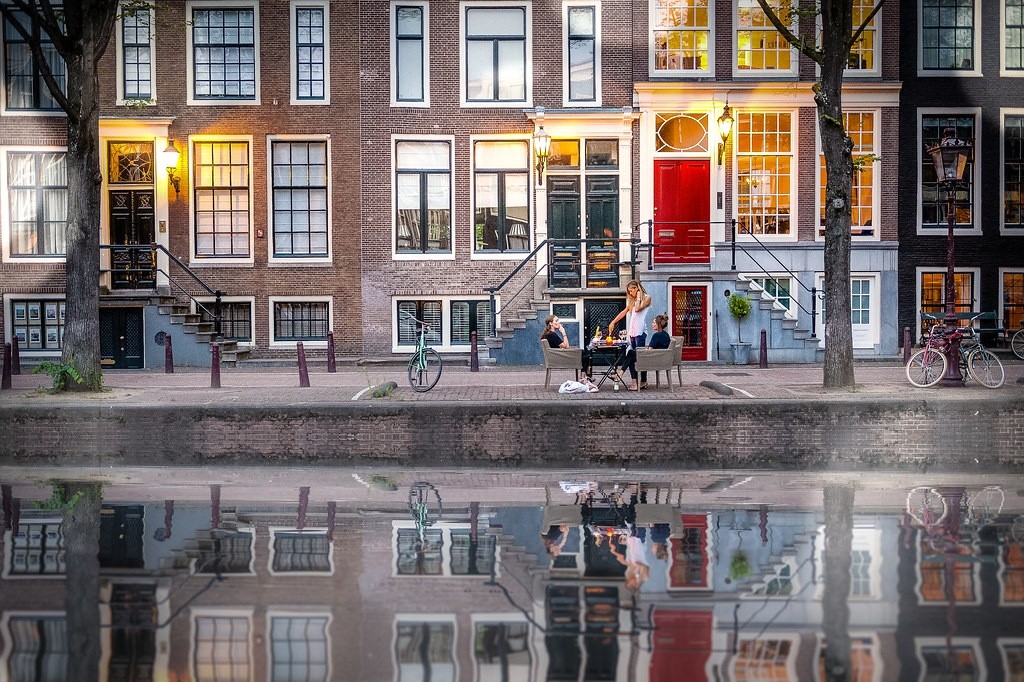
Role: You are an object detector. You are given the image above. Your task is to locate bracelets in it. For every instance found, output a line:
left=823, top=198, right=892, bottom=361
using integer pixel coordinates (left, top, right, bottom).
left=562, top=333, right=566, bottom=336
left=634, top=298, right=642, bottom=301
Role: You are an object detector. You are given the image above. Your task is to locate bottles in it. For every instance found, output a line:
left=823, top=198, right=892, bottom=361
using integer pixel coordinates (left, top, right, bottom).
left=613, top=374, right=620, bottom=393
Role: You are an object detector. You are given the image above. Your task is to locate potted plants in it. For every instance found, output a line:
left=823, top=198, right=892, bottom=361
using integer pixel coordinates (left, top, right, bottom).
left=725, top=529, right=757, bottom=579
left=726, top=293, right=753, bottom=365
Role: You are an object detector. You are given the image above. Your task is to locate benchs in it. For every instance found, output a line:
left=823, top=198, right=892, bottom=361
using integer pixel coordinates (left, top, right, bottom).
left=920, top=308, right=1008, bottom=349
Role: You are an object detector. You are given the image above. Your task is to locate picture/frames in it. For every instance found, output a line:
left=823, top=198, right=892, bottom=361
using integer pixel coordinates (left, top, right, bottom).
left=852, top=164, right=858, bottom=206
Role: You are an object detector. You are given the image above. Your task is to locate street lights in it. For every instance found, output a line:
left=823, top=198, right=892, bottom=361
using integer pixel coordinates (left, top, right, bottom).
left=927, top=126, right=972, bottom=389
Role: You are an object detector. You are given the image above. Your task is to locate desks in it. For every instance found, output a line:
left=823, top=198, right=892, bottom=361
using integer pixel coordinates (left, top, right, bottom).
left=591, top=340, right=630, bottom=391
left=590, top=482, right=629, bottom=535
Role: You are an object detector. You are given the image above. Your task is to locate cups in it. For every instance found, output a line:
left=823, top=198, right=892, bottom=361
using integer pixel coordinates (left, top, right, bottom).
left=606, top=337, right=613, bottom=345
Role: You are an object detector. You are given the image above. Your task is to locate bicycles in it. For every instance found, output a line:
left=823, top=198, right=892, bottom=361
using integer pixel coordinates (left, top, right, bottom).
left=899, top=481, right=1008, bottom=568
left=954, top=311, right=1005, bottom=390
left=907, top=309, right=949, bottom=388
left=405, top=313, right=443, bottom=392
left=400, top=481, right=453, bottom=580
left=1011, top=318, right=1024, bottom=360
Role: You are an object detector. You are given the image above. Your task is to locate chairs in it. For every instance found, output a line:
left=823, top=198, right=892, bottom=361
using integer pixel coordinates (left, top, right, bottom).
left=541, top=339, right=582, bottom=392
left=541, top=487, right=583, bottom=535
left=635, top=336, right=684, bottom=393
left=634, top=481, right=686, bottom=539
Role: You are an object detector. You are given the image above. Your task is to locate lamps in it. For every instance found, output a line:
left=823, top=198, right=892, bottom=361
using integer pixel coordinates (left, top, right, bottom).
left=162, top=137, right=181, bottom=194
left=718, top=100, right=734, bottom=165
left=746, top=157, right=763, bottom=188
left=532, top=124, right=551, bottom=185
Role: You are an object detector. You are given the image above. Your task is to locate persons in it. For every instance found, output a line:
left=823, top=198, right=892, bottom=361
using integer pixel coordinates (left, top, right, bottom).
left=541, top=481, right=671, bottom=592
left=608, top=279, right=652, bottom=391
left=540, top=311, right=601, bottom=383
left=607, top=311, right=671, bottom=391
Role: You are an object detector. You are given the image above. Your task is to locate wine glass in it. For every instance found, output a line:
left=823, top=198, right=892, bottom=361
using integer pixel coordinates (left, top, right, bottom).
left=622, top=330, right=626, bottom=345
left=619, top=331, right=624, bottom=344
left=597, top=331, right=602, bottom=345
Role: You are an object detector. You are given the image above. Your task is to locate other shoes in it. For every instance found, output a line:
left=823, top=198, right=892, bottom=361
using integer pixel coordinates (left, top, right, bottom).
left=607, top=374, right=621, bottom=382
left=640, top=382, right=648, bottom=390
left=627, top=387, right=637, bottom=391
